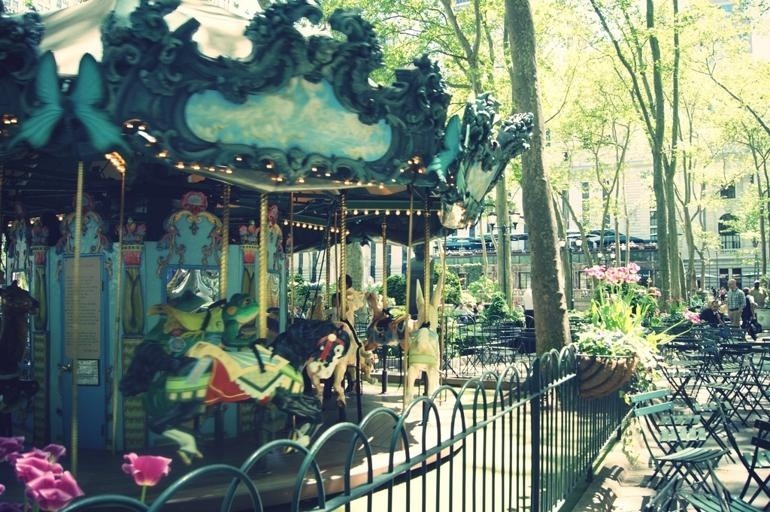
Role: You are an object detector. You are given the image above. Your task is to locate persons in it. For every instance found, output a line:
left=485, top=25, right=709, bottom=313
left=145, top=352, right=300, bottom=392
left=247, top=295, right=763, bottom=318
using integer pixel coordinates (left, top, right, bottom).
left=345, top=271, right=355, bottom=315
left=723, top=276, right=747, bottom=330
left=742, top=284, right=752, bottom=320
left=751, top=279, right=769, bottom=307
left=699, top=300, right=725, bottom=329
left=330, top=292, right=341, bottom=319
left=719, top=286, right=726, bottom=299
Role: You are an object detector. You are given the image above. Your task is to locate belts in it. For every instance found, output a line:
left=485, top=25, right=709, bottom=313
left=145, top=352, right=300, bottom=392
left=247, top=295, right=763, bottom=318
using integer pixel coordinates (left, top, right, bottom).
left=729, top=307, right=739, bottom=311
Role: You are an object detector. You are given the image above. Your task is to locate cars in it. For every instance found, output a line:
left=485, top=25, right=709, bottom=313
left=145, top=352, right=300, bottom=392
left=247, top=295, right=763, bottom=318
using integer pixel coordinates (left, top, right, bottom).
left=443, top=228, right=657, bottom=252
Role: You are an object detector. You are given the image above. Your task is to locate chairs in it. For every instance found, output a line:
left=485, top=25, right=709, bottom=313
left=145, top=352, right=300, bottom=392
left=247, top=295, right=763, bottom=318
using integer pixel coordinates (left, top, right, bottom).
left=354, top=315, right=534, bottom=376
left=628, top=311, right=770, bottom=512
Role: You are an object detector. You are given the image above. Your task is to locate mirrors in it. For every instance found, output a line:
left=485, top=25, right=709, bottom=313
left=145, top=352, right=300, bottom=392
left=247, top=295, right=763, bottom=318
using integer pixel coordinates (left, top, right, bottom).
left=161, top=263, right=220, bottom=305
left=266, top=269, right=281, bottom=310
